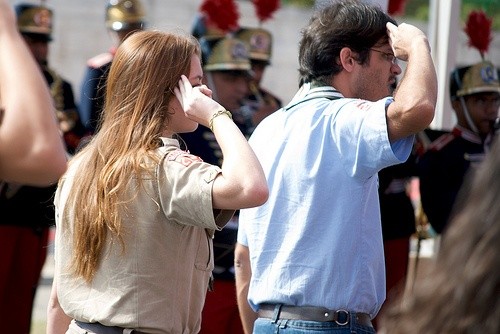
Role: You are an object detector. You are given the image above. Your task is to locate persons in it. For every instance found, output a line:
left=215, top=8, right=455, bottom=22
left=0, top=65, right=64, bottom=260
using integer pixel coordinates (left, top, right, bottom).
left=0, top=0, right=500, bottom=334
left=45, top=30, right=269, bottom=334
left=234, top=0, right=438, bottom=334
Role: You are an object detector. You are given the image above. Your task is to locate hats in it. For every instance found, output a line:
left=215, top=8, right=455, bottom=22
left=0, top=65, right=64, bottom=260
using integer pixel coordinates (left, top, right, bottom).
left=202, top=0, right=251, bottom=71
left=236, top=0, right=278, bottom=63
left=106, top=0, right=146, bottom=27
left=450, top=10, right=500, bottom=100
left=14, top=3, right=53, bottom=38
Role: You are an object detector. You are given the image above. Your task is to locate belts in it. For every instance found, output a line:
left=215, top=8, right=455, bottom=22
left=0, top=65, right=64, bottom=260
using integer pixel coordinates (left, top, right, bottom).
left=75, top=319, right=149, bottom=334
left=258, top=304, right=373, bottom=327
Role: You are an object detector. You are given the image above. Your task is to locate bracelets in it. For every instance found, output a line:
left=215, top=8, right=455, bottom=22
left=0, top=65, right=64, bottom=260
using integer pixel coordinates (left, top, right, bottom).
left=209, top=110, right=232, bottom=135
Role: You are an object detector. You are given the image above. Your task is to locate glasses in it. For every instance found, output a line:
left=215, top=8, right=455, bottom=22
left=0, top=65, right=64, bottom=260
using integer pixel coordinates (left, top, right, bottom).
left=362, top=46, right=397, bottom=65
